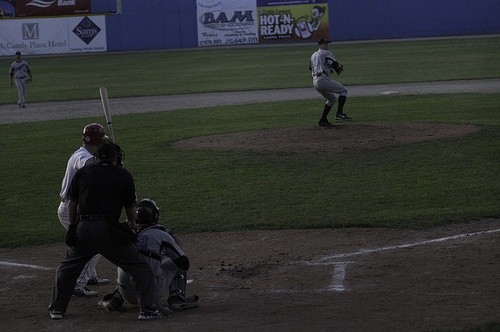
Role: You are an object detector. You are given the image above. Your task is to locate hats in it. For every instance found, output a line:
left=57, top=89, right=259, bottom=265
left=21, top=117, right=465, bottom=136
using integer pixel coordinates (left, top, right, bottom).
left=318, top=38, right=331, bottom=44
left=16, top=51, right=21, bottom=55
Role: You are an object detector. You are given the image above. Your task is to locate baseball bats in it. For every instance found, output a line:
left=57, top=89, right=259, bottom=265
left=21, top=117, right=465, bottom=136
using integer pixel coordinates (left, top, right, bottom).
left=99, top=87, right=116, bottom=146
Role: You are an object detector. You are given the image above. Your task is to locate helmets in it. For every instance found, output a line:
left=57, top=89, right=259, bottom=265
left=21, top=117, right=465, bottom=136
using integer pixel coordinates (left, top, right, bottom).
left=135, top=199, right=160, bottom=223
left=96, top=143, right=121, bottom=164
left=82, top=123, right=109, bottom=145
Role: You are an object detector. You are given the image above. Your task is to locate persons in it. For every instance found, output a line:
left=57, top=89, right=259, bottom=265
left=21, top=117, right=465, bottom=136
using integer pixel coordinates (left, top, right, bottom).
left=9, top=51, right=32, bottom=108
left=47, top=143, right=173, bottom=319
left=98, top=199, right=199, bottom=310
left=309, top=38, right=352, bottom=126
left=57, top=123, right=113, bottom=298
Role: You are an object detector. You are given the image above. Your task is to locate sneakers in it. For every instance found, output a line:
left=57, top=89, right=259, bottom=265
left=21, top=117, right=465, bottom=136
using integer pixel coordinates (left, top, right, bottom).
left=72, top=287, right=97, bottom=297
left=50, top=307, right=62, bottom=319
left=138, top=306, right=173, bottom=319
left=168, top=295, right=200, bottom=308
left=97, top=290, right=124, bottom=312
left=336, top=114, right=352, bottom=121
left=317, top=120, right=333, bottom=127
left=87, top=276, right=110, bottom=285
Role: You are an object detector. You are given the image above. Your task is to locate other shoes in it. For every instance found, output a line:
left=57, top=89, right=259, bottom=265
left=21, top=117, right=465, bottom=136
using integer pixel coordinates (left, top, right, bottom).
left=17, top=104, right=25, bottom=108
